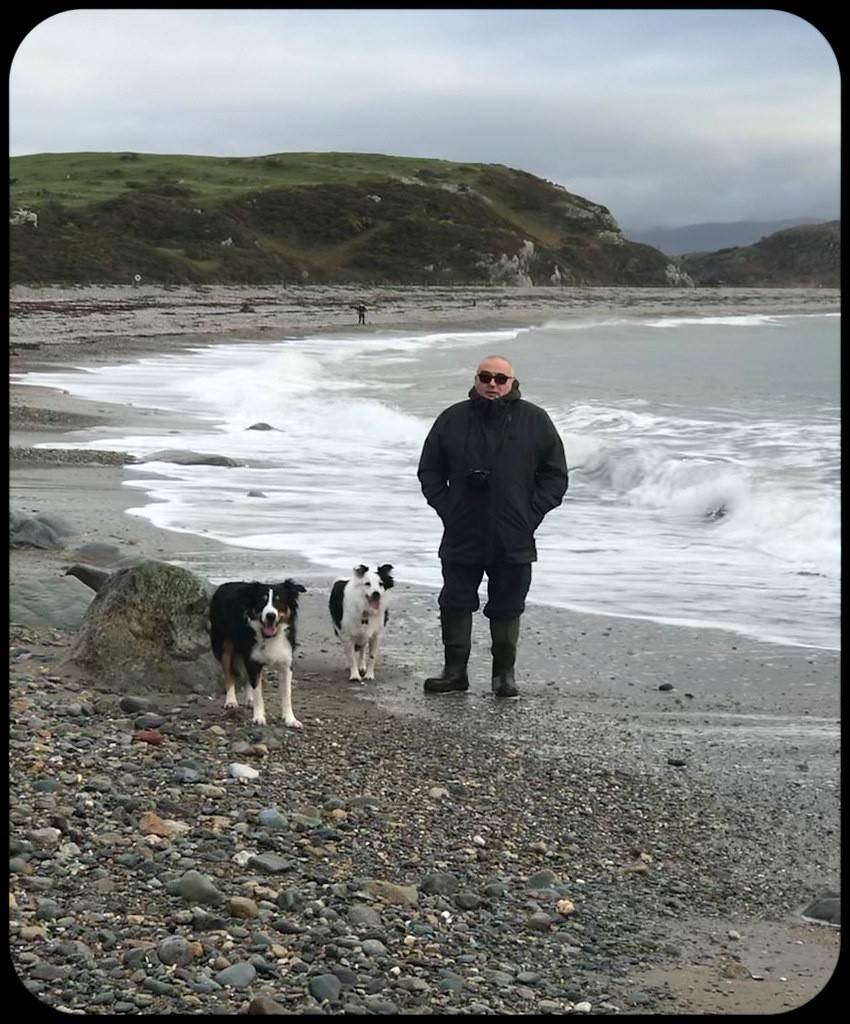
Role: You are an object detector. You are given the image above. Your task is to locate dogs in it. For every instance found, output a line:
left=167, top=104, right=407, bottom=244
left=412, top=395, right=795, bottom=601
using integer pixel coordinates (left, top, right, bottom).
left=210, top=578, right=307, bottom=729
left=327, top=563, right=395, bottom=679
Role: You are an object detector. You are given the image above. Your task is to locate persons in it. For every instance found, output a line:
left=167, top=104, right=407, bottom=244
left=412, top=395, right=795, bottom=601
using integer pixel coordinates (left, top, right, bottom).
left=416, top=355, right=569, bottom=698
left=356, top=303, right=368, bottom=325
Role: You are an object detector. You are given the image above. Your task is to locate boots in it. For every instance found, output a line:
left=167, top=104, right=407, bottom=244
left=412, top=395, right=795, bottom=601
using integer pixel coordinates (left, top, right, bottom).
left=490, top=618, right=520, bottom=698
left=424, top=613, right=473, bottom=691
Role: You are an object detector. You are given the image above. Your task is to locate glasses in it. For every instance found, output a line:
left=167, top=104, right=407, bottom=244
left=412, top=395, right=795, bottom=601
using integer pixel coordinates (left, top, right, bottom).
left=475, top=371, right=512, bottom=384
